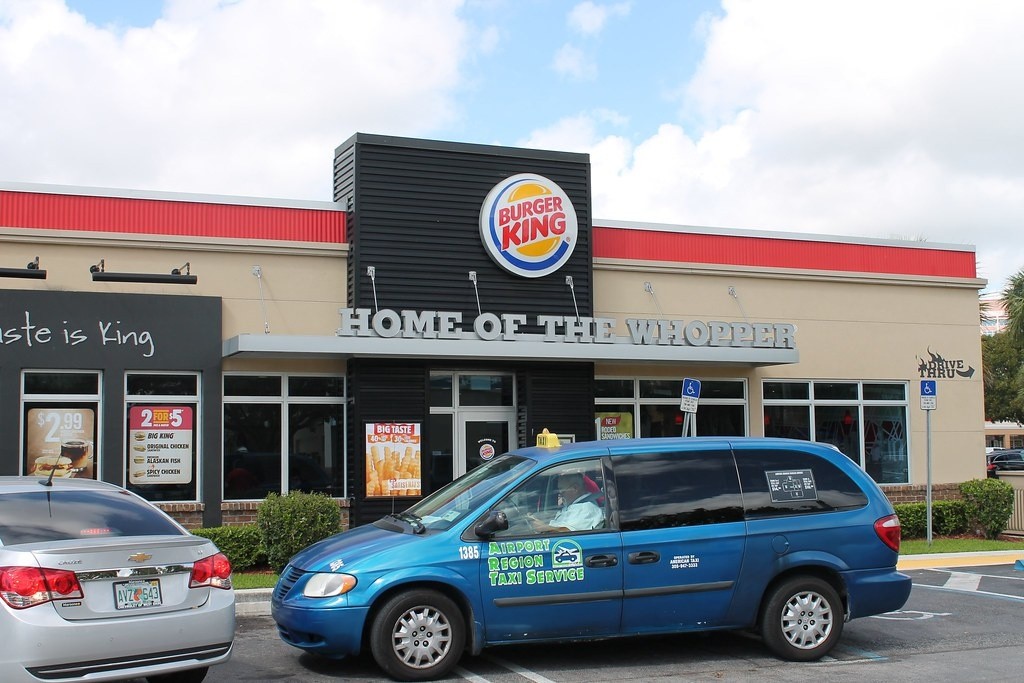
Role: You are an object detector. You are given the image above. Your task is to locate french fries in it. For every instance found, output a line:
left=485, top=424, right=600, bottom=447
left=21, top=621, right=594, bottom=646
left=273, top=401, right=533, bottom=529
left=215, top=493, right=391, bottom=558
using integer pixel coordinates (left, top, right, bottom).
left=365, top=446, right=421, bottom=496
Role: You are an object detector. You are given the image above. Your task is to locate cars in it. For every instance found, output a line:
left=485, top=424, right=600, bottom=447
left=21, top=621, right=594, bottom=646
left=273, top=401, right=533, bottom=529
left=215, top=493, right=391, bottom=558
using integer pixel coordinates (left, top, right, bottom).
left=270, top=427, right=912, bottom=682
left=986, top=447, right=1024, bottom=470
left=0, top=476, right=236, bottom=683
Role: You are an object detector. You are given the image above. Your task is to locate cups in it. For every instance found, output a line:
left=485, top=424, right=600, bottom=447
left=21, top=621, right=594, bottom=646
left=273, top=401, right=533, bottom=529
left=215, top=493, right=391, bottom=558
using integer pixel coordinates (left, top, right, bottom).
left=61, top=438, right=93, bottom=473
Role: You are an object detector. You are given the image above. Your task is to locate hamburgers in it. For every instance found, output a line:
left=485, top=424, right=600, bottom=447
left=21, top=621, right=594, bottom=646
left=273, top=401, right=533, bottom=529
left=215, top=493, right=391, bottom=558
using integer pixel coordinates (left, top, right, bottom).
left=133, top=431, right=146, bottom=477
left=34, top=456, right=72, bottom=479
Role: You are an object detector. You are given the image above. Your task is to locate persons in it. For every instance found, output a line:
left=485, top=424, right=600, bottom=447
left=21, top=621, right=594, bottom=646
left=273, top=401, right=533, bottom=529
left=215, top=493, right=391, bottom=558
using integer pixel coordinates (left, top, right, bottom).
left=527, top=472, right=603, bottom=534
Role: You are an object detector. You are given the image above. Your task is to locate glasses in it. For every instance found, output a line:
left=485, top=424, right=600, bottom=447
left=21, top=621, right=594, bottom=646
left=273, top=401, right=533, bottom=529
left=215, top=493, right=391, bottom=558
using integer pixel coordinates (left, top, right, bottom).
left=556, top=483, right=575, bottom=497
